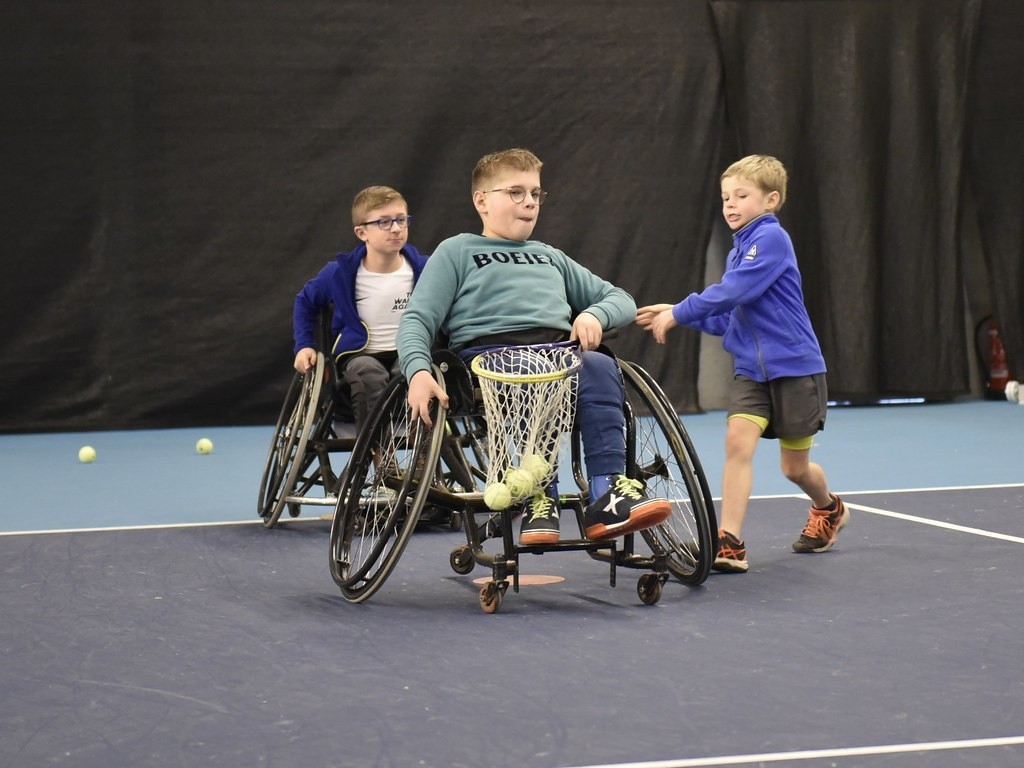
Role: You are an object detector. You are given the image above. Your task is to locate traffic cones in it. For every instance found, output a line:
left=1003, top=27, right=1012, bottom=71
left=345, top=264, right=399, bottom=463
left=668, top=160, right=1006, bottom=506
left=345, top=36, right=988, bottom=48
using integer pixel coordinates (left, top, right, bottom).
left=986, top=321, right=1016, bottom=399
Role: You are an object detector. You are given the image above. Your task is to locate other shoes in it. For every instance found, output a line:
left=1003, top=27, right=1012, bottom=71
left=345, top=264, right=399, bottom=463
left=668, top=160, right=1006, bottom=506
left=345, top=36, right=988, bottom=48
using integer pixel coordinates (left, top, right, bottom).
left=373, top=446, right=403, bottom=496
left=409, top=442, right=447, bottom=493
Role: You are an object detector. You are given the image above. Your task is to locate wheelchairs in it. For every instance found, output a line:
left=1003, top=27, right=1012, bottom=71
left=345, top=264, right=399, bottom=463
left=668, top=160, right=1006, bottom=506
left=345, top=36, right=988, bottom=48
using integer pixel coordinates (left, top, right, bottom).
left=329, top=316, right=722, bottom=612
left=258, top=348, right=507, bottom=531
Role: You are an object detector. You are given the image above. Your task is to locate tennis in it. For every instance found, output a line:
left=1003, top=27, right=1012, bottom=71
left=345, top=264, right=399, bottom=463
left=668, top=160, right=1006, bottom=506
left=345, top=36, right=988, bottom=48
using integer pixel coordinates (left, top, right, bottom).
left=77, top=444, right=97, bottom=463
left=483, top=482, right=511, bottom=510
left=195, top=437, right=213, bottom=456
left=508, top=470, right=532, bottom=500
left=524, top=454, right=549, bottom=481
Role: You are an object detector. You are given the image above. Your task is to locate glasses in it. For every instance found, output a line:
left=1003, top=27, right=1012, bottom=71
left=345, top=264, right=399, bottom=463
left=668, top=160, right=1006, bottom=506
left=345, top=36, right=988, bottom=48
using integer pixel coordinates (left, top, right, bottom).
left=482, top=186, right=549, bottom=206
left=358, top=216, right=412, bottom=231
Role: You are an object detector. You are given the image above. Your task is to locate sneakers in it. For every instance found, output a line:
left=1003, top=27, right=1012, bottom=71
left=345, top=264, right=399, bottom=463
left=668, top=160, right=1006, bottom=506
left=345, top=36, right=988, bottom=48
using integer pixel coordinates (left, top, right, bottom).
left=793, top=495, right=850, bottom=553
left=585, top=474, right=673, bottom=540
left=677, top=529, right=751, bottom=573
left=519, top=488, right=560, bottom=547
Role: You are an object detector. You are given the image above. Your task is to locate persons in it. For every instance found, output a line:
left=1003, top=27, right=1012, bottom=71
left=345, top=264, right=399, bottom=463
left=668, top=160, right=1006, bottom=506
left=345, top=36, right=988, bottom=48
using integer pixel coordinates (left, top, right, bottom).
left=635, top=153, right=852, bottom=573
left=394, top=147, right=672, bottom=546
left=293, top=185, right=452, bottom=500
